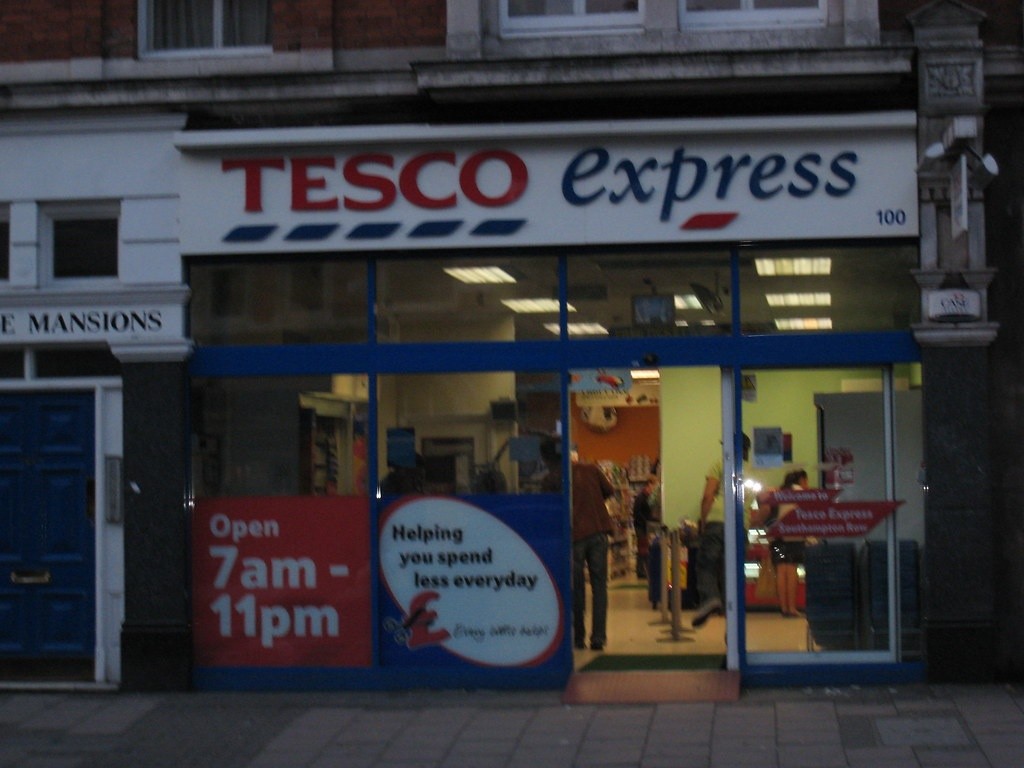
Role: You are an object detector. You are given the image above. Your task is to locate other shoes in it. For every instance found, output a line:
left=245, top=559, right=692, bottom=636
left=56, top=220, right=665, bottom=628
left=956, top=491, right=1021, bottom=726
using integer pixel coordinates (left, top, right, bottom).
left=575, top=643, right=586, bottom=648
left=692, top=598, right=722, bottom=626
left=590, top=645, right=603, bottom=649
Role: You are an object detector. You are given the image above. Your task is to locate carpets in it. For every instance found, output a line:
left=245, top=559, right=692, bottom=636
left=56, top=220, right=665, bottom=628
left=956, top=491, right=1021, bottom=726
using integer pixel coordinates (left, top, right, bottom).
left=576, top=653, right=726, bottom=670
left=560, top=670, right=742, bottom=704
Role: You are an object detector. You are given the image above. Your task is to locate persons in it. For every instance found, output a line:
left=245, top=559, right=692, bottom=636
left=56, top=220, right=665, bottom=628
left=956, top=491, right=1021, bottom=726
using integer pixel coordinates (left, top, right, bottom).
left=540, top=441, right=615, bottom=651
left=764, top=469, right=810, bottom=617
left=633, top=479, right=660, bottom=580
left=692, top=432, right=771, bottom=626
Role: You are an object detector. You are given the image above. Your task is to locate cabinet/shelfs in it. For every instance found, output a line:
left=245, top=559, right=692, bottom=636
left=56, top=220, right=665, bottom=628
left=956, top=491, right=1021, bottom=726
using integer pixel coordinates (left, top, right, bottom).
left=606, top=487, right=631, bottom=583
left=629, top=475, right=661, bottom=570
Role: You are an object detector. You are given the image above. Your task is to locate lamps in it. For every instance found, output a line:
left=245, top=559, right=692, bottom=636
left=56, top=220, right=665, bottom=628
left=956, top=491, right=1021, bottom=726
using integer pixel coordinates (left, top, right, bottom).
left=690, top=270, right=723, bottom=318
